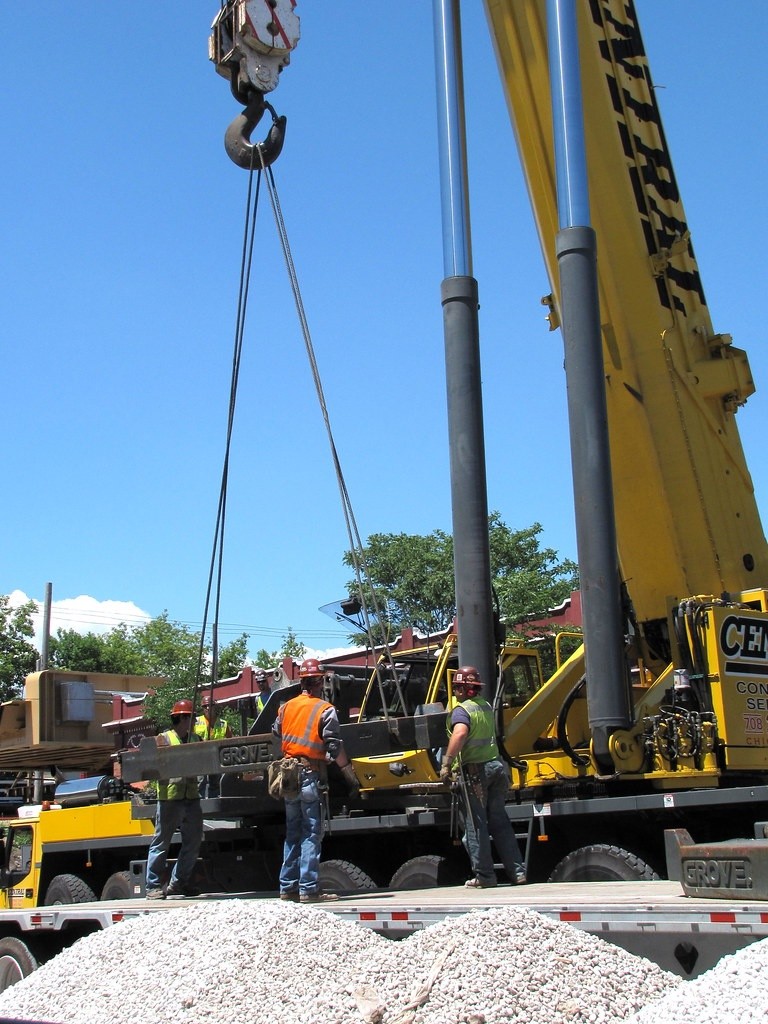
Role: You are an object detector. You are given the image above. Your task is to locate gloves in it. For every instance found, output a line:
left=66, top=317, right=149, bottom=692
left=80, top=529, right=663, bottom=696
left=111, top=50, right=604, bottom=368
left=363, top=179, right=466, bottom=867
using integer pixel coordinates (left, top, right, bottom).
left=439, top=755, right=452, bottom=786
left=341, top=764, right=359, bottom=799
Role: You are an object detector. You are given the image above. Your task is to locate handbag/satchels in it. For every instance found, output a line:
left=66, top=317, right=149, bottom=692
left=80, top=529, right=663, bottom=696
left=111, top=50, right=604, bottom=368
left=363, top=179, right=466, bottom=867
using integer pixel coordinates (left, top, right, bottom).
left=268, top=757, right=301, bottom=798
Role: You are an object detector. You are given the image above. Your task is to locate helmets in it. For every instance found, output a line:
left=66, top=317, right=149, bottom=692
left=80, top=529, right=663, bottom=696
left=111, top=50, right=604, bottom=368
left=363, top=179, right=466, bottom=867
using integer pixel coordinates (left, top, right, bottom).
left=170, top=700, right=196, bottom=716
left=201, top=696, right=217, bottom=706
left=452, top=667, right=487, bottom=686
left=299, top=659, right=324, bottom=677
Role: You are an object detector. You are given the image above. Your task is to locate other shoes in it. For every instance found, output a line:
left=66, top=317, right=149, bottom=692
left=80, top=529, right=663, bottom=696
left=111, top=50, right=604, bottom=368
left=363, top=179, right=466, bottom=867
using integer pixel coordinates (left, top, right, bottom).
left=465, top=878, right=482, bottom=888
left=504, top=870, right=526, bottom=885
left=167, top=887, right=200, bottom=896
left=300, top=893, right=338, bottom=902
left=146, top=890, right=165, bottom=899
left=281, top=894, right=299, bottom=899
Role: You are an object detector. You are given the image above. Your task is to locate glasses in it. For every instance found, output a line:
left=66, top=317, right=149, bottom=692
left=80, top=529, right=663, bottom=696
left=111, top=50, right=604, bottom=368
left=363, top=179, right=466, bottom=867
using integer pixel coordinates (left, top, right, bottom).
left=184, top=716, right=195, bottom=719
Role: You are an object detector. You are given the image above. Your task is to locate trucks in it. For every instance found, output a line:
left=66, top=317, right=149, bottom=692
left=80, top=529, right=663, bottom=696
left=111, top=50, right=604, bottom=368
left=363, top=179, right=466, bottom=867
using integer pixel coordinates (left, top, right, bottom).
left=0, top=580, right=768, bottom=989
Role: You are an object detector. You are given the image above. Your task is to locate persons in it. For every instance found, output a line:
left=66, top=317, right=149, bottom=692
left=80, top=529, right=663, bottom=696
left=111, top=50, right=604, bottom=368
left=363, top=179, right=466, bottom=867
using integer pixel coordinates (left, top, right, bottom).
left=145, top=701, right=205, bottom=900
left=194, top=695, right=232, bottom=798
left=270, top=659, right=360, bottom=903
left=440, top=664, right=526, bottom=888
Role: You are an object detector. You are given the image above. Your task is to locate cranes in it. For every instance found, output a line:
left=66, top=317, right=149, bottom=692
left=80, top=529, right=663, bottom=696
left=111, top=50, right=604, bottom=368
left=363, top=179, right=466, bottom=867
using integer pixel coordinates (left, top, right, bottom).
left=208, top=0, right=768, bottom=801
